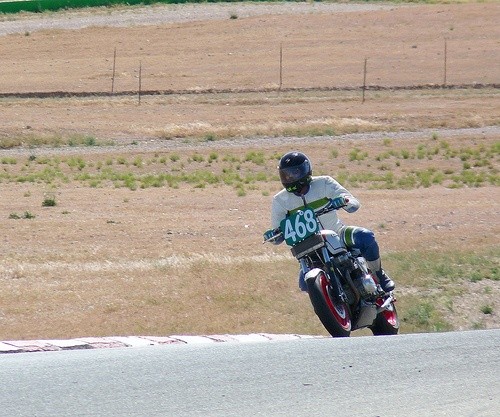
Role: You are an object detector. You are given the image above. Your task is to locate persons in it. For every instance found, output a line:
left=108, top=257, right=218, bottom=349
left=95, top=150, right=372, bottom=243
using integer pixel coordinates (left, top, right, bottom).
left=263, top=151, right=395, bottom=292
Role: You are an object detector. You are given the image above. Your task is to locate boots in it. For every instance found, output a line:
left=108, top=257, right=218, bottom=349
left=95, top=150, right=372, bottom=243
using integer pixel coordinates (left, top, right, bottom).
left=366, top=257, right=395, bottom=292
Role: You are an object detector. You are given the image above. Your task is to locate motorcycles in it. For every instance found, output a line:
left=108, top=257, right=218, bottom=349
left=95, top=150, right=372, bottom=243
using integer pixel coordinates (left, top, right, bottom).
left=261, top=198, right=400, bottom=338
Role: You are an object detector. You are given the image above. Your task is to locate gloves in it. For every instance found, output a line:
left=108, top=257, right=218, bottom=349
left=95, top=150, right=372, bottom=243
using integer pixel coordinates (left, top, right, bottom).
left=263, top=228, right=276, bottom=241
left=332, top=195, right=347, bottom=210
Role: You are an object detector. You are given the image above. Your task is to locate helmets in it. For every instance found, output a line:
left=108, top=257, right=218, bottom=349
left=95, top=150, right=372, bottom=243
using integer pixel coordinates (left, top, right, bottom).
left=279, top=152, right=312, bottom=188
